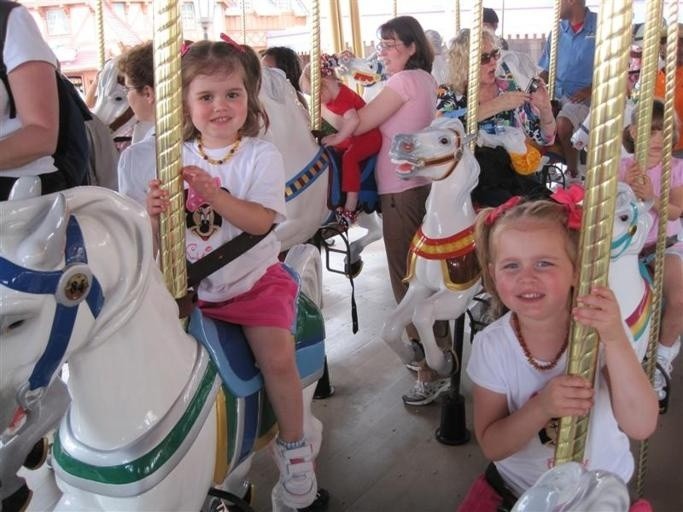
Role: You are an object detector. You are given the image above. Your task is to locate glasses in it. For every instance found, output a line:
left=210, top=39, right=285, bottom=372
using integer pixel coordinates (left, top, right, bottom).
left=481, top=49, right=500, bottom=65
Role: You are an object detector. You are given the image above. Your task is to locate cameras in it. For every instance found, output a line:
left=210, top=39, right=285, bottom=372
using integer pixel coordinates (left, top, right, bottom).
left=525, top=77, right=539, bottom=94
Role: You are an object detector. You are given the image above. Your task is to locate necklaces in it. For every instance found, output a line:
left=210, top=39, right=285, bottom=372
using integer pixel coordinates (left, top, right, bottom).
left=196, top=133, right=241, bottom=164
left=510, top=312, right=570, bottom=372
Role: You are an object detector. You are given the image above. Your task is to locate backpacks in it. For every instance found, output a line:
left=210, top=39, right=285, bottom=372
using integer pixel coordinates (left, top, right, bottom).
left=51, top=73, right=121, bottom=191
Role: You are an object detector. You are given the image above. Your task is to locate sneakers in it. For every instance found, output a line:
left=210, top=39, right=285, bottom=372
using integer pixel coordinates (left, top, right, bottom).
left=544, top=168, right=587, bottom=197
left=652, top=346, right=674, bottom=399
left=401, top=367, right=452, bottom=406
left=271, top=438, right=319, bottom=509
left=404, top=358, right=426, bottom=371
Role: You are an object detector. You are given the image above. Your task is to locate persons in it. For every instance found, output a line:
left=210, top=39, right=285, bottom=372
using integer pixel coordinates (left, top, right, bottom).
left=256, top=5, right=683, bottom=235
left=143, top=34, right=317, bottom=508
left=612, top=98, right=682, bottom=413
left=454, top=191, right=659, bottom=510
left=0, top=1, right=92, bottom=202
left=318, top=15, right=456, bottom=404
left=539, top=1, right=599, bottom=184
left=114, top=38, right=159, bottom=207
